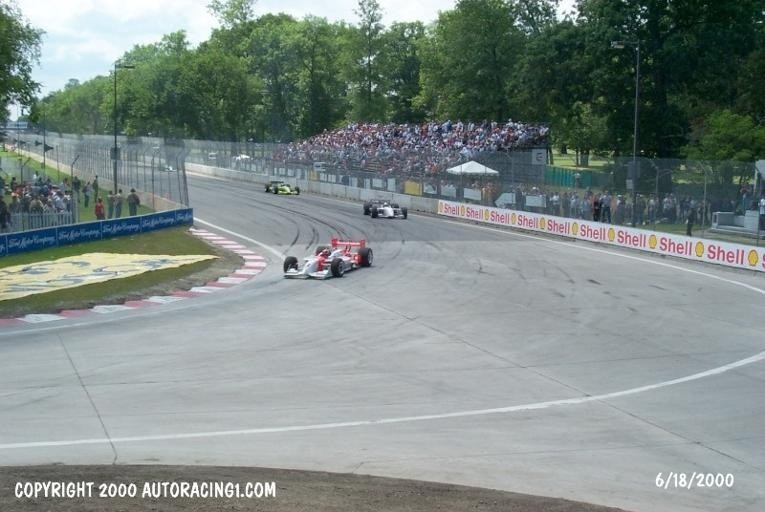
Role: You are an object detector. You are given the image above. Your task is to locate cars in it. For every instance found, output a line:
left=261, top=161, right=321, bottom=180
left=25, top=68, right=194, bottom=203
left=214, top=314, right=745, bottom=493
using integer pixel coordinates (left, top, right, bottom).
left=283, top=238, right=375, bottom=283
left=208, top=153, right=251, bottom=169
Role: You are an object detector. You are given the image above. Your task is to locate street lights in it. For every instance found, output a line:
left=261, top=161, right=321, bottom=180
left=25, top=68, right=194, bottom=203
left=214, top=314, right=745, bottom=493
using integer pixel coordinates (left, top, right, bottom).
left=16, top=104, right=29, bottom=157
left=612, top=37, right=642, bottom=227
left=112, top=62, right=137, bottom=195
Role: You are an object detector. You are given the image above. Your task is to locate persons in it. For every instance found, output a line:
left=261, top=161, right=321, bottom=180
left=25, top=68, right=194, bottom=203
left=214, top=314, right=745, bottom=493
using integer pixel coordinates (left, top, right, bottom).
left=0, top=167, right=142, bottom=233
left=267, top=118, right=765, bottom=238
